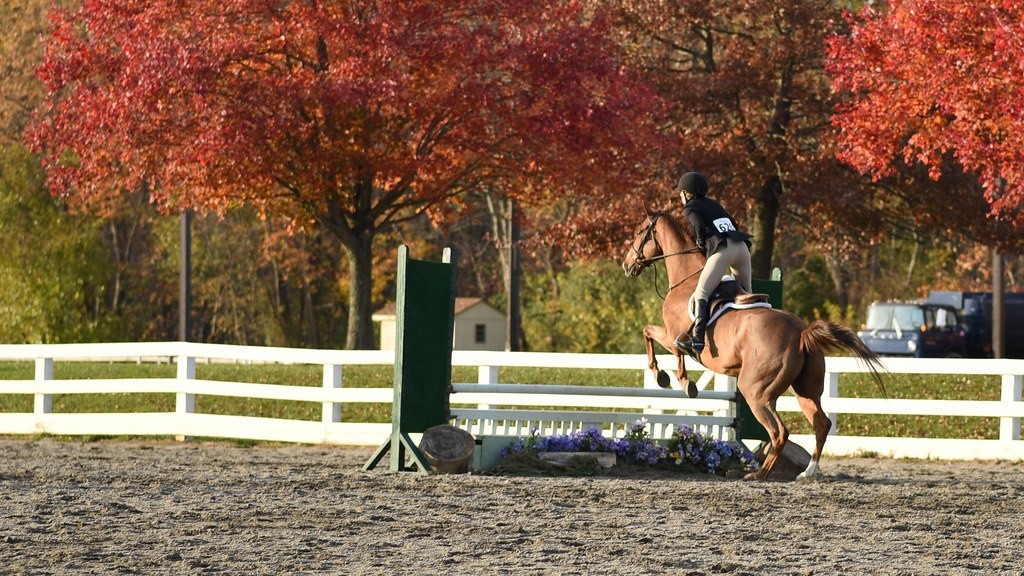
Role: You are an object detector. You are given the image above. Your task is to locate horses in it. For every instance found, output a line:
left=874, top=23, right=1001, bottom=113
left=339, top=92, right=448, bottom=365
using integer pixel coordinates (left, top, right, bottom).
left=621, top=199, right=899, bottom=485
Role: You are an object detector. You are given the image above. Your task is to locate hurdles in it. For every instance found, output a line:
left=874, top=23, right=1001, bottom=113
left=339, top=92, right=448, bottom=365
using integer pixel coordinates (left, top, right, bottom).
left=357, top=241, right=783, bottom=475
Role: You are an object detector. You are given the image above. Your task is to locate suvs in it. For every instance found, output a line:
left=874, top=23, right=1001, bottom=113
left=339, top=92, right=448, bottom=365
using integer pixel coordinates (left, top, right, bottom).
left=856, top=299, right=969, bottom=358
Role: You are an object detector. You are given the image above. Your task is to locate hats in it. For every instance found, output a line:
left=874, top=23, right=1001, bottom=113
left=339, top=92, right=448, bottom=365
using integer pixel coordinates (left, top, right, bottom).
left=678, top=172, right=708, bottom=196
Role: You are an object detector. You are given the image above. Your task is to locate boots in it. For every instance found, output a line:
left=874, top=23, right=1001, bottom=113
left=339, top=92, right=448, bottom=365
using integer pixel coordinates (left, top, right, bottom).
left=676, top=299, right=710, bottom=354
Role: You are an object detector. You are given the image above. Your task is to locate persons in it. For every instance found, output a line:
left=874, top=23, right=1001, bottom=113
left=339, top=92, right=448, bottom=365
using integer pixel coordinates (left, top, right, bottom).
left=676, top=172, right=752, bottom=354
